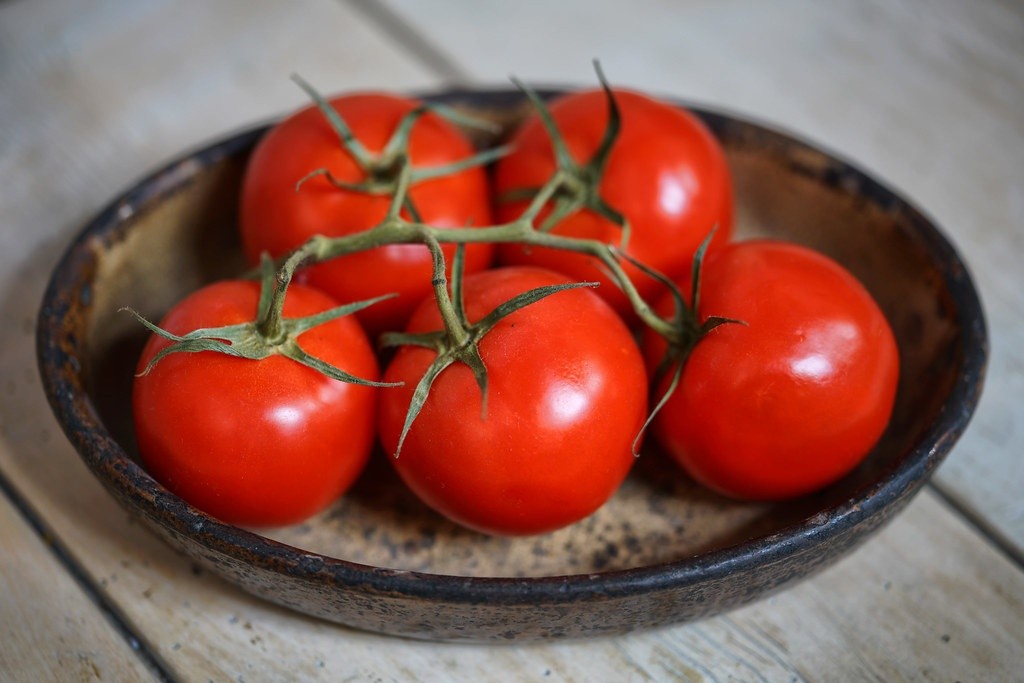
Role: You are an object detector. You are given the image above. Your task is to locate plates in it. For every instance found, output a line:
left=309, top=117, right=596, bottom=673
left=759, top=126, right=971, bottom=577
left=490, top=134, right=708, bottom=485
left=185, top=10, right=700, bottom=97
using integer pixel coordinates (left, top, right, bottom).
left=32, top=83, right=989, bottom=645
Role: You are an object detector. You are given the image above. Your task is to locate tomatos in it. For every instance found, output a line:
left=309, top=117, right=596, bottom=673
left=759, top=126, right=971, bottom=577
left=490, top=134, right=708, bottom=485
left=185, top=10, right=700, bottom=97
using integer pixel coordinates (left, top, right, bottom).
left=116, top=50, right=899, bottom=538
